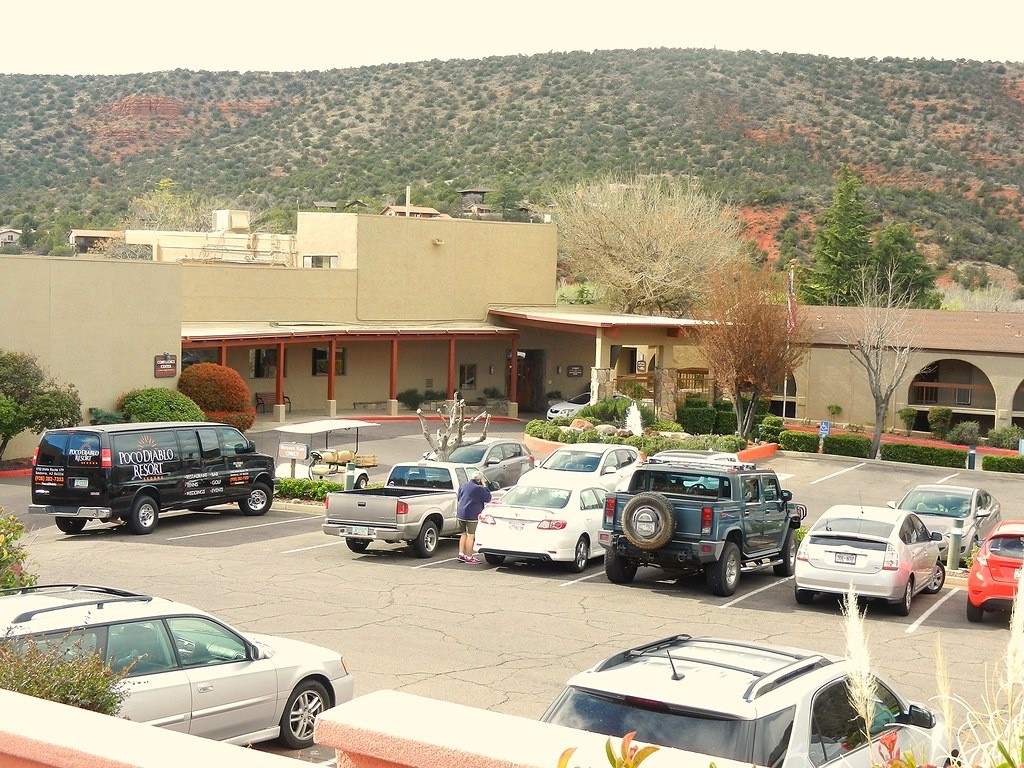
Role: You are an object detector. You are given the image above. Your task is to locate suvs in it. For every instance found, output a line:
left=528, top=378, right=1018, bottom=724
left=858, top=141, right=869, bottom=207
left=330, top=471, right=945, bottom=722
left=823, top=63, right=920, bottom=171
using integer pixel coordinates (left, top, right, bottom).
left=598, top=458, right=807, bottom=597
left=0, top=584, right=351, bottom=749
left=536, top=634, right=945, bottom=768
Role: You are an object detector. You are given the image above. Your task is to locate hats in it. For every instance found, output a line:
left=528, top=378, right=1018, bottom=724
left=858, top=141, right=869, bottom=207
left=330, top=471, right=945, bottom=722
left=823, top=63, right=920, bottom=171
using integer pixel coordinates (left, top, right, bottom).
left=470, top=471, right=483, bottom=480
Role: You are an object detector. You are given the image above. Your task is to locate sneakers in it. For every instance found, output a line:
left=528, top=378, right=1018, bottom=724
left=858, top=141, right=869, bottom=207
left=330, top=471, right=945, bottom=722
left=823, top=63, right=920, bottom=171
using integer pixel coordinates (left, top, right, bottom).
left=457, top=552, right=482, bottom=565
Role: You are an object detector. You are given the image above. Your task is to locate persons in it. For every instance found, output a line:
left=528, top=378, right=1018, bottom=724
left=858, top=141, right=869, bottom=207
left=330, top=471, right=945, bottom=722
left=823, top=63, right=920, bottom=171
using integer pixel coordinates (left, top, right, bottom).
left=456, top=471, right=491, bottom=564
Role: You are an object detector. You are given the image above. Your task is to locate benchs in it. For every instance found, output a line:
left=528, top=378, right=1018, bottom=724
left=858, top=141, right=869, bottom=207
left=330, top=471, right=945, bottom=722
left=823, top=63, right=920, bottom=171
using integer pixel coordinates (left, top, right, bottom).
left=353, top=401, right=387, bottom=409
left=255, top=393, right=292, bottom=414
left=312, top=450, right=352, bottom=478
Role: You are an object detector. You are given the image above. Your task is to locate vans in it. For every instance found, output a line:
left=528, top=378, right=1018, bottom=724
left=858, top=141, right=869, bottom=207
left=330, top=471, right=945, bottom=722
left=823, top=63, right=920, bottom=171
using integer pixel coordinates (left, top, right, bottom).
left=28, top=423, right=279, bottom=535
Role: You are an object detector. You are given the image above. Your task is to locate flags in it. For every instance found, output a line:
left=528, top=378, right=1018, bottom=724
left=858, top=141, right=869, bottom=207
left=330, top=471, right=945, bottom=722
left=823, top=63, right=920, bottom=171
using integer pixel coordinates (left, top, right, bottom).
left=787, top=280, right=798, bottom=336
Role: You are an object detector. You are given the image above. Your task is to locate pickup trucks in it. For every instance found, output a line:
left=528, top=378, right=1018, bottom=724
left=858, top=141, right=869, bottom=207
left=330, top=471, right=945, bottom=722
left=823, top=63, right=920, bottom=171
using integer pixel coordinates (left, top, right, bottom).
left=322, top=461, right=503, bottom=559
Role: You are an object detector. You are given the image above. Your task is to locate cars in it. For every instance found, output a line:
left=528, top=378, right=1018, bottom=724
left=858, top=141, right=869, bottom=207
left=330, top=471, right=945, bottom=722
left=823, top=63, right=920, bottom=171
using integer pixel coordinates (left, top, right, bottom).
left=419, top=438, right=535, bottom=490
left=651, top=448, right=741, bottom=463
left=886, top=483, right=1001, bottom=566
left=795, top=506, right=946, bottom=615
left=546, top=392, right=635, bottom=419
left=967, top=520, right=1024, bottom=622
left=517, top=443, right=647, bottom=488
left=473, top=481, right=611, bottom=573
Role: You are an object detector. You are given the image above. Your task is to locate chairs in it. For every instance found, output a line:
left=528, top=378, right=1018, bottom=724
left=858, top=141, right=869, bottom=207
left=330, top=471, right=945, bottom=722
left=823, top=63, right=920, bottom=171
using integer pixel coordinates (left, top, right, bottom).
left=917, top=503, right=938, bottom=511
